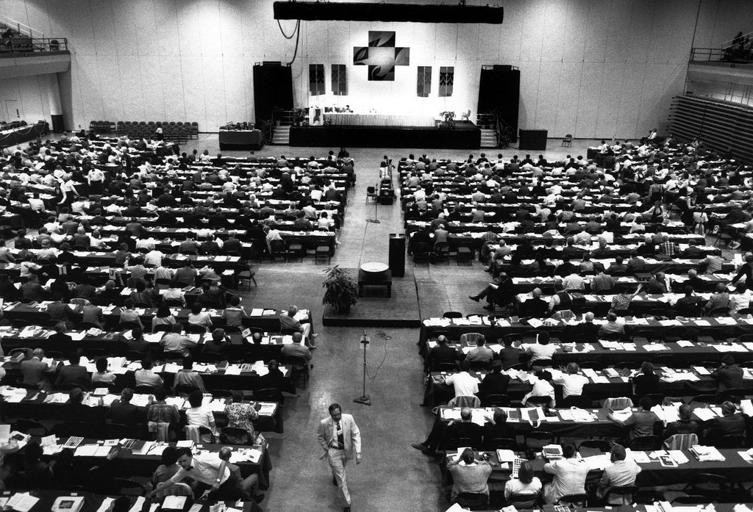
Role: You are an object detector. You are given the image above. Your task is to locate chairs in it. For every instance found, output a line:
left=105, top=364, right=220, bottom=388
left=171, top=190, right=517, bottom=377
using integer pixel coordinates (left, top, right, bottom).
left=0, top=119, right=358, bottom=512
left=0, top=23, right=37, bottom=56
left=562, top=133, right=572, bottom=147
left=399, top=136, right=752, bottom=511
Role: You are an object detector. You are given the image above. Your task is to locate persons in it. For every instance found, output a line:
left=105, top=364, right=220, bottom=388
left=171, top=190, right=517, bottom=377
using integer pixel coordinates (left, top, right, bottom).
left=317, top=403, right=361, bottom=512
left=720, top=31, right=753, bottom=61
left=2, top=122, right=356, bottom=512
left=293, top=103, right=354, bottom=126
left=2, top=27, right=16, bottom=49
left=378, top=128, right=752, bottom=512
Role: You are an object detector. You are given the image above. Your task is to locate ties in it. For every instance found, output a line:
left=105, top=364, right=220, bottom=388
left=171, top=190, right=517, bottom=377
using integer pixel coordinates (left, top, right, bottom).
left=337, top=422, right=343, bottom=447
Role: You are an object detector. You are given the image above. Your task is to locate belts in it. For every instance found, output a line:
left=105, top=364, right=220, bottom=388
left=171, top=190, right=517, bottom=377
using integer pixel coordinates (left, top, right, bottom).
left=331, top=445, right=344, bottom=449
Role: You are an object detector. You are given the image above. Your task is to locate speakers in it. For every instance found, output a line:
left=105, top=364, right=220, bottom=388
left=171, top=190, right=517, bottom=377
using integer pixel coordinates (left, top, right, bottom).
left=390, top=233, right=405, bottom=276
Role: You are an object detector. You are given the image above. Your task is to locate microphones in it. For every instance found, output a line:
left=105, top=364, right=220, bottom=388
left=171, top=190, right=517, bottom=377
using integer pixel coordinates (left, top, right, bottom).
left=363, top=330, right=367, bottom=336
left=376, top=183, right=378, bottom=186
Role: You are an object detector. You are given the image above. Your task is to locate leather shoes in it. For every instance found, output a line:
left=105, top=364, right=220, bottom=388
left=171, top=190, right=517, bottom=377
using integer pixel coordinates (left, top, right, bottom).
left=484, top=305, right=491, bottom=309
left=344, top=507, right=350, bottom=512
left=470, top=296, right=479, bottom=302
left=312, top=333, right=318, bottom=338
left=411, top=442, right=435, bottom=456
left=309, top=344, right=316, bottom=349
left=257, top=494, right=264, bottom=504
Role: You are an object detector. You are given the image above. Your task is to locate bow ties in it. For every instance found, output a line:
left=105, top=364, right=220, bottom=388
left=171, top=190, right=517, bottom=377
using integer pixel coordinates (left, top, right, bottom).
left=187, top=466, right=193, bottom=470
